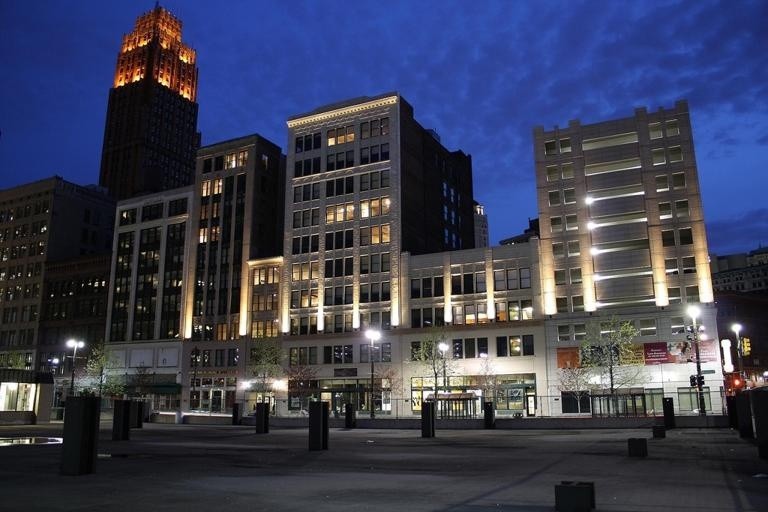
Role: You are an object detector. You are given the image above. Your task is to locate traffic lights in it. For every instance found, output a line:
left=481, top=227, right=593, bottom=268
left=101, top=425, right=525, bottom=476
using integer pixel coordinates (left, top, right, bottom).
left=726, top=375, right=733, bottom=393
left=743, top=337, right=751, bottom=356
left=732, top=372, right=741, bottom=388
left=689, top=374, right=697, bottom=386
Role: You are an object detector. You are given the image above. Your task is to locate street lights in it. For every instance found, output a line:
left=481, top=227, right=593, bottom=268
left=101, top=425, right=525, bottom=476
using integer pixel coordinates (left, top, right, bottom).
left=364, top=329, right=381, bottom=418
left=685, top=304, right=709, bottom=415
left=439, top=342, right=450, bottom=392
left=730, top=322, right=746, bottom=388
left=67, top=338, right=85, bottom=395
